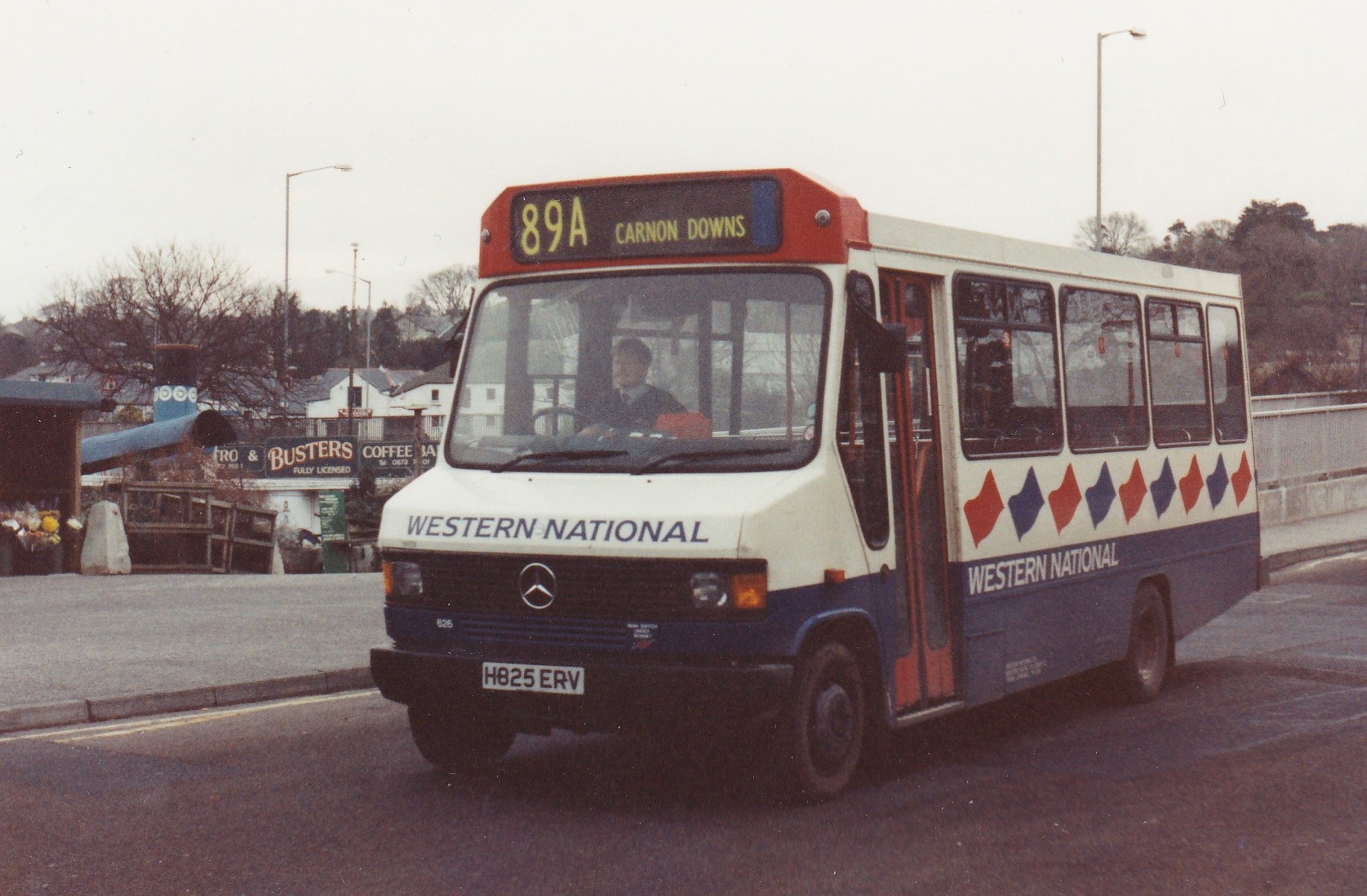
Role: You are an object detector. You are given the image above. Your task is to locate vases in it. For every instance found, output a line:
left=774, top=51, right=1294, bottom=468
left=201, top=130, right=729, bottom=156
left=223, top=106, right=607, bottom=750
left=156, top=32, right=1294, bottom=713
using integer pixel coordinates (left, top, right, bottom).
left=22, top=551, right=57, bottom=576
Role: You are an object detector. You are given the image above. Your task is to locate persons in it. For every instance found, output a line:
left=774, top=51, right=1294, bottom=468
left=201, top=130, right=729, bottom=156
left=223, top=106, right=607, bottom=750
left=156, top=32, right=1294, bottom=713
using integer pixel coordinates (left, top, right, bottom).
left=580, top=337, right=689, bottom=438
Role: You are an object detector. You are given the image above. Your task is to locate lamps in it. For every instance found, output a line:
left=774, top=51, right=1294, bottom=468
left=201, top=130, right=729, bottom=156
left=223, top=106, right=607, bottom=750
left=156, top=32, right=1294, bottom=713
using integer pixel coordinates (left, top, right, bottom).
left=99, top=398, right=119, bottom=413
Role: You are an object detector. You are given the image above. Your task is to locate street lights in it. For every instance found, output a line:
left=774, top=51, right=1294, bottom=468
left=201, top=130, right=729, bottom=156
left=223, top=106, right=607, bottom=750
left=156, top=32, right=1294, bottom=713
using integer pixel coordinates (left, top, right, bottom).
left=1097, top=29, right=1150, bottom=253
left=325, top=269, right=371, bottom=439
left=284, top=165, right=353, bottom=369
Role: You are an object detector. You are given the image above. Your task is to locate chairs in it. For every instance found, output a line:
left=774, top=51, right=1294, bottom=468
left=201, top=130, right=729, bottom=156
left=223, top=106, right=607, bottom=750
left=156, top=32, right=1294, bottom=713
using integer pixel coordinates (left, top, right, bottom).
left=827, top=415, right=1226, bottom=463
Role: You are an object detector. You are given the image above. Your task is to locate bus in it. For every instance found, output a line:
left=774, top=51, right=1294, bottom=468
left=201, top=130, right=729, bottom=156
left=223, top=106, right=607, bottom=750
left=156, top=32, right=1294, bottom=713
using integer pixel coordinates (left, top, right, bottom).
left=368, top=168, right=1272, bottom=807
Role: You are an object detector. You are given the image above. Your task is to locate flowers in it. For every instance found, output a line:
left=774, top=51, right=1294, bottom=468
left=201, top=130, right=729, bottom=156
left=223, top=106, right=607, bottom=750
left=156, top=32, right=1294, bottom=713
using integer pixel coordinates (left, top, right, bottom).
left=13, top=519, right=62, bottom=548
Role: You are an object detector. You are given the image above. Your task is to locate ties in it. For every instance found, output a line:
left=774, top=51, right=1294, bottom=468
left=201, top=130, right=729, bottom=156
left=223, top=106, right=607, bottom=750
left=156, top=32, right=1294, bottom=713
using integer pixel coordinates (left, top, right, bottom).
left=621, top=393, right=630, bottom=406
